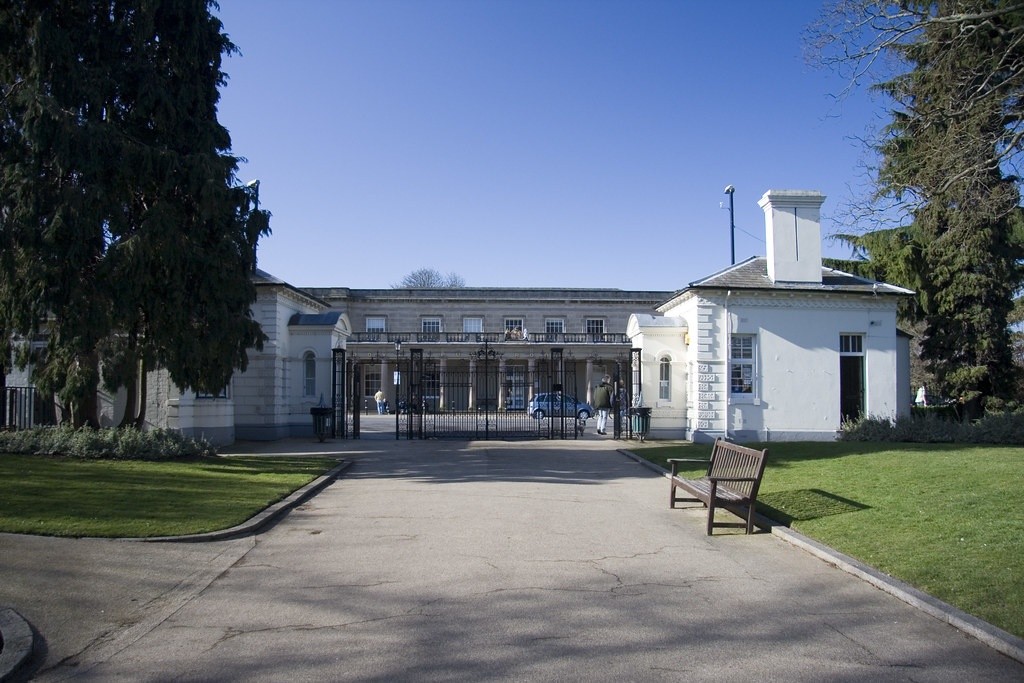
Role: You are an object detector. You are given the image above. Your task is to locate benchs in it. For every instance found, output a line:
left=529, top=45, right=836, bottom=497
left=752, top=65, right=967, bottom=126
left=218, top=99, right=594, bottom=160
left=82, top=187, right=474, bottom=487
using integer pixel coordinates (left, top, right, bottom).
left=667, top=436, right=768, bottom=535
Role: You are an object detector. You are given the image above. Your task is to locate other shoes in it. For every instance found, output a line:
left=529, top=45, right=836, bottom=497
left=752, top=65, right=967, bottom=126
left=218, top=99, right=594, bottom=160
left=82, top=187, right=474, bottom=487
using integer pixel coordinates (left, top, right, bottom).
left=597, top=429, right=607, bottom=435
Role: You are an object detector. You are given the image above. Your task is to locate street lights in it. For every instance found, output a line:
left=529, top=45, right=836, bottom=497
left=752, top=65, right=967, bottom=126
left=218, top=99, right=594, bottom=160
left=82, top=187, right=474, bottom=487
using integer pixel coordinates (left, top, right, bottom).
left=719, top=185, right=735, bottom=265
left=247, top=178, right=262, bottom=273
left=394, top=339, right=402, bottom=439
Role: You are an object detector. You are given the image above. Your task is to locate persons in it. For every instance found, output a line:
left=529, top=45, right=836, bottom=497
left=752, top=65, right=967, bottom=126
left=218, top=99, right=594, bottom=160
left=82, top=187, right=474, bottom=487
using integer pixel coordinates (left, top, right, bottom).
left=593, top=374, right=614, bottom=435
left=374, top=389, right=384, bottom=415
left=610, top=380, right=627, bottom=433
left=383, top=399, right=390, bottom=415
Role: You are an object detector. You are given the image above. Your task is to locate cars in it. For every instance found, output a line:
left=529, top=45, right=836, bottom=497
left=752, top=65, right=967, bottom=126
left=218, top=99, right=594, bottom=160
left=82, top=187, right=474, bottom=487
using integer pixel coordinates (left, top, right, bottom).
left=911, top=395, right=947, bottom=406
left=527, top=393, right=595, bottom=419
